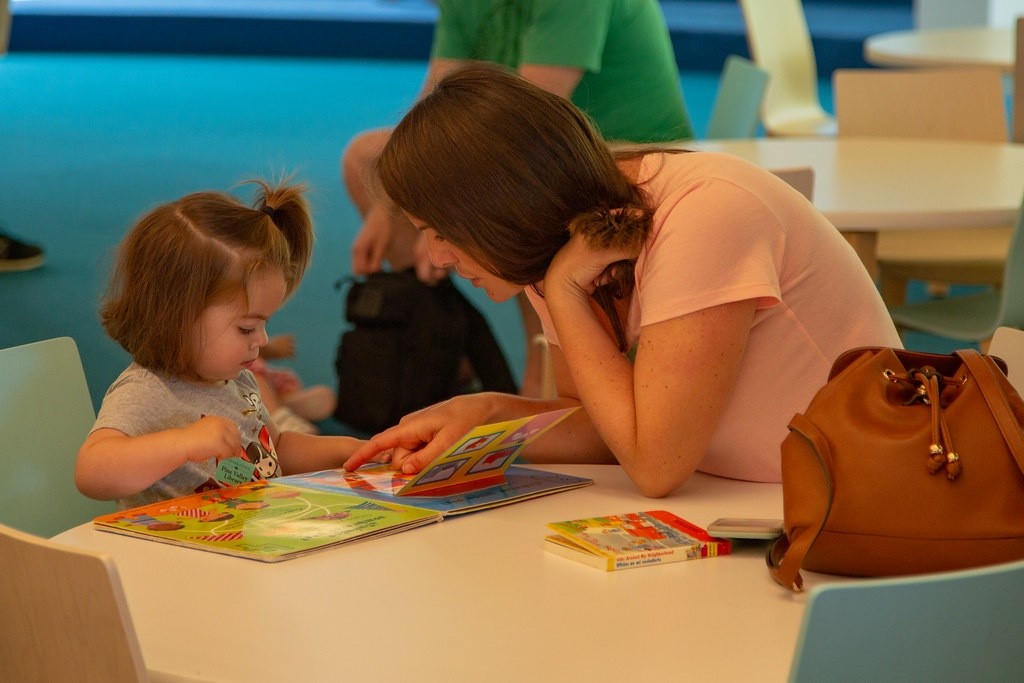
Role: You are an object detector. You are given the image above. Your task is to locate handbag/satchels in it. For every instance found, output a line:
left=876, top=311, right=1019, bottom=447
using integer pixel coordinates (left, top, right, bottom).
left=334, top=266, right=520, bottom=440
left=764, top=345, right=1024, bottom=595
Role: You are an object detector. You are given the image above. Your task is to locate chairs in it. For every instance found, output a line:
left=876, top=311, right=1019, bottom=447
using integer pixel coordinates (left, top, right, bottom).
left=0, top=338, right=117, bottom=539
left=0, top=524, right=154, bottom=683
left=785, top=562, right=1024, bottom=683
left=826, top=66, right=1011, bottom=305
left=701, top=56, right=771, bottom=139
left=740, top=0, right=846, bottom=136
left=886, top=191, right=1024, bottom=356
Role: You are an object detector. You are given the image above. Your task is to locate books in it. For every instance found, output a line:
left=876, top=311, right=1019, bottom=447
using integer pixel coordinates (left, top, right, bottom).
left=544, top=510, right=732, bottom=572
left=92, top=404, right=594, bottom=561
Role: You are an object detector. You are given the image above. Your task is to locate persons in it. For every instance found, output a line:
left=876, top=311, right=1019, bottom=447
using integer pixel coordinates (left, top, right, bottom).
left=246, top=334, right=337, bottom=435
left=345, top=60, right=905, bottom=500
left=343, top=0, right=698, bottom=283
left=75, top=167, right=396, bottom=510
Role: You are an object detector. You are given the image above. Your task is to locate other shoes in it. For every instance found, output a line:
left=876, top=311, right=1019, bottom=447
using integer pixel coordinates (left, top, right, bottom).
left=0, top=233, right=45, bottom=271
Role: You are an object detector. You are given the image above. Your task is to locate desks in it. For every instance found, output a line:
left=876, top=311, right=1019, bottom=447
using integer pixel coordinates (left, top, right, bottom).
left=48, top=465, right=1024, bottom=683
left=614, top=134, right=1024, bottom=291
left=861, top=19, right=1024, bottom=64
